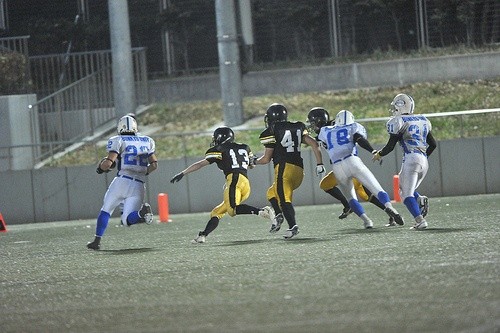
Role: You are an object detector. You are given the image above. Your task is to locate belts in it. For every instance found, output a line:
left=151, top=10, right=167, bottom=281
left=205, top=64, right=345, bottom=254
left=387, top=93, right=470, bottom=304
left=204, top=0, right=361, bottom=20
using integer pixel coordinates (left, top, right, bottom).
left=116, top=174, right=145, bottom=184
left=404, top=150, right=428, bottom=157
left=333, top=154, right=357, bottom=164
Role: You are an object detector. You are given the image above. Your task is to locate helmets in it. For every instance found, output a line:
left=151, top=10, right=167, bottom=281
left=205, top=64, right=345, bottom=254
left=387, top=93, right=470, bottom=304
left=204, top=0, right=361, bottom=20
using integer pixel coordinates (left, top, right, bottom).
left=265, top=103, right=288, bottom=122
left=213, top=127, right=235, bottom=144
left=117, top=116, right=138, bottom=133
left=391, top=94, right=414, bottom=116
left=335, top=110, right=354, bottom=125
left=306, top=108, right=330, bottom=128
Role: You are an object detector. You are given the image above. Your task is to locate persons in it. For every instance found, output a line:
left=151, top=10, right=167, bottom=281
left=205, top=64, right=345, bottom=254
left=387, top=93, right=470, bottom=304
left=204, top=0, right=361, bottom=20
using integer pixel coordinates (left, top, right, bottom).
left=250, top=104, right=327, bottom=240
left=170, top=126, right=278, bottom=244
left=303, top=106, right=405, bottom=229
left=372, top=94, right=437, bottom=230
left=86, top=112, right=159, bottom=250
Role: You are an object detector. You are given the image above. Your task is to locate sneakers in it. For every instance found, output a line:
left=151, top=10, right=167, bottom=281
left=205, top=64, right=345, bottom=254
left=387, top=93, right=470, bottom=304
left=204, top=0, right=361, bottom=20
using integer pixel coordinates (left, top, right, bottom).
left=410, top=219, right=428, bottom=230
left=385, top=206, right=405, bottom=225
left=338, top=208, right=354, bottom=219
left=191, top=231, right=205, bottom=243
left=142, top=203, right=153, bottom=225
left=419, top=196, right=429, bottom=218
left=385, top=218, right=396, bottom=226
left=269, top=213, right=284, bottom=233
left=87, top=241, right=100, bottom=250
left=283, top=225, right=300, bottom=239
left=363, top=219, right=374, bottom=229
left=258, top=206, right=275, bottom=221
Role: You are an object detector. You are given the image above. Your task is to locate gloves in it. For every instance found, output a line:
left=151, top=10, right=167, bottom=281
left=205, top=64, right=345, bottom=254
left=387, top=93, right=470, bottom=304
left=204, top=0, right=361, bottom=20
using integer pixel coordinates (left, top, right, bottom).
left=316, top=164, right=326, bottom=176
left=170, top=172, right=184, bottom=184
left=249, top=156, right=258, bottom=167
left=372, top=150, right=382, bottom=162
